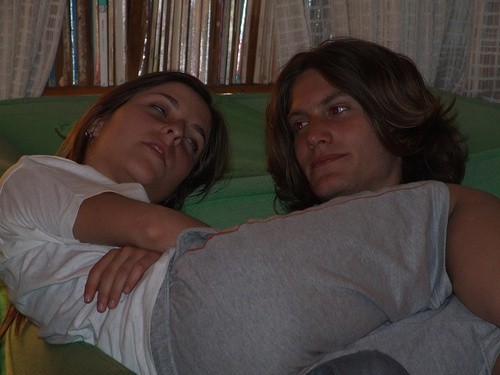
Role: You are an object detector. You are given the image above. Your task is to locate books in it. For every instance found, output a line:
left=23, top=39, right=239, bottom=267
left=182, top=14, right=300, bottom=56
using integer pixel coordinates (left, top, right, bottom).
left=46, top=0, right=259, bottom=86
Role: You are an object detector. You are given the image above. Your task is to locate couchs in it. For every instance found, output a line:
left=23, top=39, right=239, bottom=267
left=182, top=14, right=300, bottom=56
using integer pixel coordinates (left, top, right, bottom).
left=0, top=82, right=499, bottom=375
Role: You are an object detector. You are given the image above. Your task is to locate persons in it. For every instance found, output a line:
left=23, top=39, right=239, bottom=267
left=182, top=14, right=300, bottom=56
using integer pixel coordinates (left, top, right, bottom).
left=265, top=37, right=470, bottom=212
left=1, top=71, right=500, bottom=374
